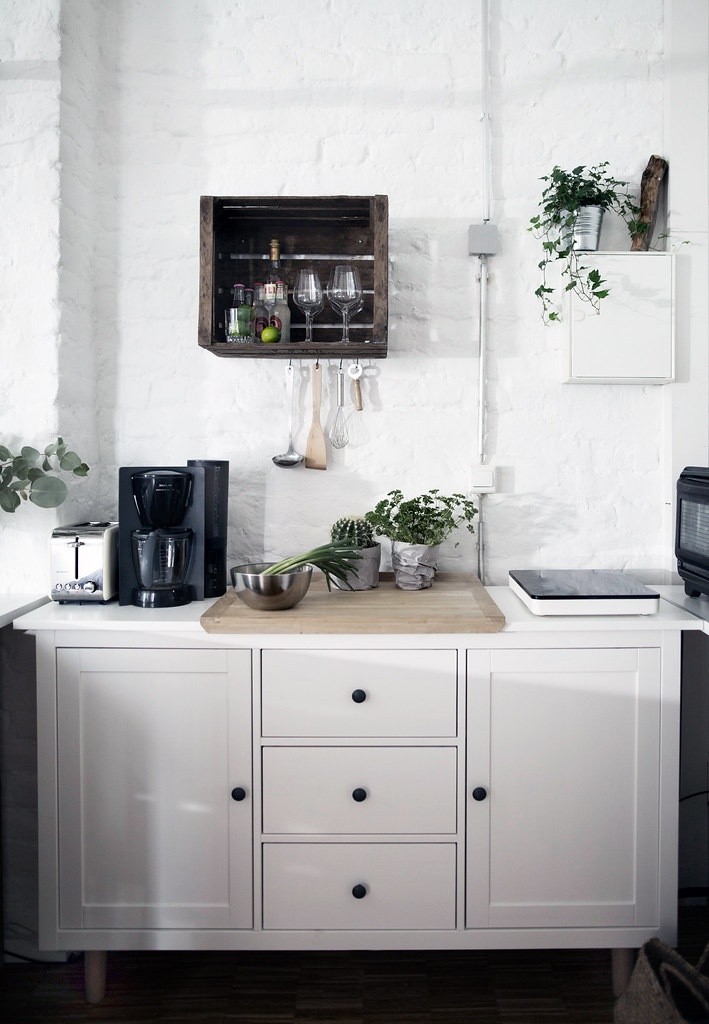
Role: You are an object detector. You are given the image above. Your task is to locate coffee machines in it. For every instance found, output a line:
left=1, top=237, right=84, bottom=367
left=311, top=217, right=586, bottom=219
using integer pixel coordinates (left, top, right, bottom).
left=118, top=460, right=228, bottom=607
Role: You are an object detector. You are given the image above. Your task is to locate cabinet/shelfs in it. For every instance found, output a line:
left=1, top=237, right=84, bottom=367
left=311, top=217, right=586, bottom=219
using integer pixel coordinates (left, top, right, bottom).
left=22, top=628, right=680, bottom=1001
left=564, top=252, right=678, bottom=386
left=199, top=193, right=392, bottom=359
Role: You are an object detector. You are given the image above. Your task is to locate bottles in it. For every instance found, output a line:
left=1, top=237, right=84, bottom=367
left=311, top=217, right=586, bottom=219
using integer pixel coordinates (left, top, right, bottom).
left=223, top=284, right=256, bottom=343
left=254, top=283, right=269, bottom=343
left=243, top=288, right=254, bottom=306
left=263, top=239, right=286, bottom=285
left=269, top=281, right=290, bottom=344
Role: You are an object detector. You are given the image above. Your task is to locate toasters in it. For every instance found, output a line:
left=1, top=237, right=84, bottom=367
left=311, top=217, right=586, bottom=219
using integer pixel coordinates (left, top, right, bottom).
left=48, top=521, right=120, bottom=604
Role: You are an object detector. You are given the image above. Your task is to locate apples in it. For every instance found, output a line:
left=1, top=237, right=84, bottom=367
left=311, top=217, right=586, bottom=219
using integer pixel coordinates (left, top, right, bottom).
left=227, top=304, right=254, bottom=336
left=261, top=327, right=282, bottom=343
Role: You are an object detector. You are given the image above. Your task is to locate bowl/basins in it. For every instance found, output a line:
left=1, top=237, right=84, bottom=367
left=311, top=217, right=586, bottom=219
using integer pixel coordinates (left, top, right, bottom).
left=230, top=561, right=313, bottom=611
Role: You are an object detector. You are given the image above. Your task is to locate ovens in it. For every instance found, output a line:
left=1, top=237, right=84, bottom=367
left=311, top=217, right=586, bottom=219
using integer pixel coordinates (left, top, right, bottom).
left=674, top=465, right=708, bottom=604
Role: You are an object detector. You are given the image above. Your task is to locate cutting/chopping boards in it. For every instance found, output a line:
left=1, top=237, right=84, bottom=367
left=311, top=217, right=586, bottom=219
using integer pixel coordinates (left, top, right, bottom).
left=200, top=572, right=507, bottom=634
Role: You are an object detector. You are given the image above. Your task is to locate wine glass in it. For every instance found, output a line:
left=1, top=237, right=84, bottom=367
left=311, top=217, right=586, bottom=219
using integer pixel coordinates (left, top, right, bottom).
left=292, top=266, right=365, bottom=342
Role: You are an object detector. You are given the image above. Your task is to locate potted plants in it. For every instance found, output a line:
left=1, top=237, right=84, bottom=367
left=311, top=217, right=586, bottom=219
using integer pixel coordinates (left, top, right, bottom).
left=329, top=488, right=485, bottom=589
left=526, top=160, right=695, bottom=325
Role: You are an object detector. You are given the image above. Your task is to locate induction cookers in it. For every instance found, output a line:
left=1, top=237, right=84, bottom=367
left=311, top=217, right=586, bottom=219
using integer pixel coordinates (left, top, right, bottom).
left=509, top=569, right=660, bottom=617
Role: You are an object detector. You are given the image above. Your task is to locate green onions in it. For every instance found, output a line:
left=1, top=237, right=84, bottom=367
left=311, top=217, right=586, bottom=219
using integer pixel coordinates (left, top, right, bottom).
left=258, top=537, right=364, bottom=592
